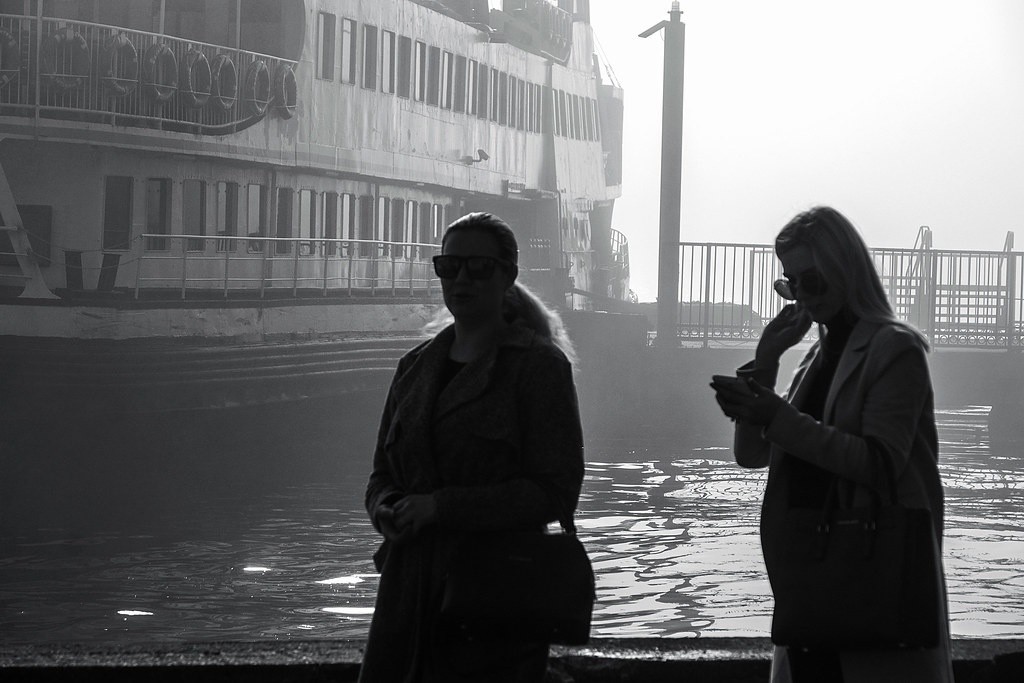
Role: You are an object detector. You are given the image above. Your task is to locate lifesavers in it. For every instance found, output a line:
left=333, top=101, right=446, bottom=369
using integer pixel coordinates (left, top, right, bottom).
left=0, top=28, right=21, bottom=88
left=209, top=54, right=238, bottom=109
left=243, top=60, right=271, bottom=117
left=181, top=50, right=213, bottom=107
left=40, top=29, right=92, bottom=94
left=99, top=34, right=139, bottom=97
left=276, top=65, right=298, bottom=120
left=141, top=43, right=179, bottom=103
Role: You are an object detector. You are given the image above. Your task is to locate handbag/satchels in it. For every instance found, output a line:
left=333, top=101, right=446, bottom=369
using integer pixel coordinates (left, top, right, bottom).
left=770, top=433, right=921, bottom=653
left=438, top=470, right=593, bottom=646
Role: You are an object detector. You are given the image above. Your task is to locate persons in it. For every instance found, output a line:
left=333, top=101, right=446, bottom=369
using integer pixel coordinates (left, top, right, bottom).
left=708, top=206, right=952, bottom=683
left=360, top=212, right=596, bottom=683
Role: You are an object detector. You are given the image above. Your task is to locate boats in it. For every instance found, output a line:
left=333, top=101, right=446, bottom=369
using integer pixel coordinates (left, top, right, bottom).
left=0, top=1, right=628, bottom=430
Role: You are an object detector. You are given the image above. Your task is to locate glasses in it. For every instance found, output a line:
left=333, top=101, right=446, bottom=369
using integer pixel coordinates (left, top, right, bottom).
left=775, top=267, right=830, bottom=298
left=433, top=255, right=510, bottom=280
